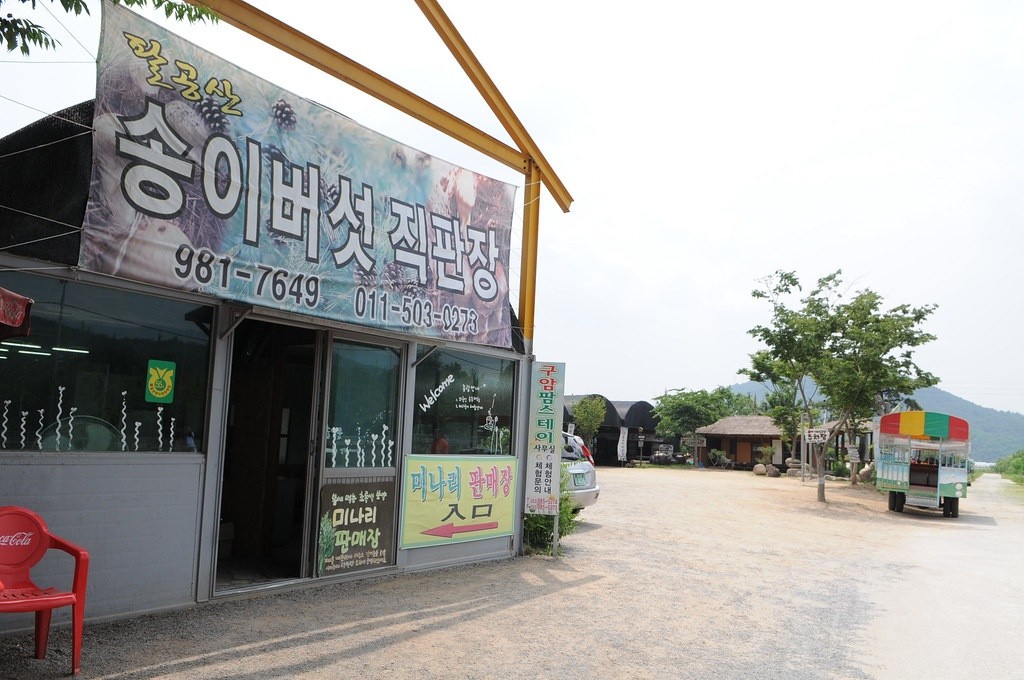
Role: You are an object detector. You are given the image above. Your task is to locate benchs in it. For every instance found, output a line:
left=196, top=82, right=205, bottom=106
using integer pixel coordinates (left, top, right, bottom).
left=910, top=464, right=938, bottom=487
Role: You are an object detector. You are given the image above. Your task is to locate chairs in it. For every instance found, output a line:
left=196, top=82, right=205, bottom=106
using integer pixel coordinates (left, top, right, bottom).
left=0, top=505, right=90, bottom=675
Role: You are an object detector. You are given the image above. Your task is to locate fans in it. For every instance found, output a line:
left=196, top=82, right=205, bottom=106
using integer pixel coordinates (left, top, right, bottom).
left=31, top=415, right=130, bottom=452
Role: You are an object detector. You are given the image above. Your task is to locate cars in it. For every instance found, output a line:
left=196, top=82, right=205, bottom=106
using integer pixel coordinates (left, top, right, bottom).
left=561, top=431, right=600, bottom=513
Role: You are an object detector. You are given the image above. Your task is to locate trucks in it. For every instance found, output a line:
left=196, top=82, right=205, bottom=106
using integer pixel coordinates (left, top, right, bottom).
left=876, top=411, right=971, bottom=517
